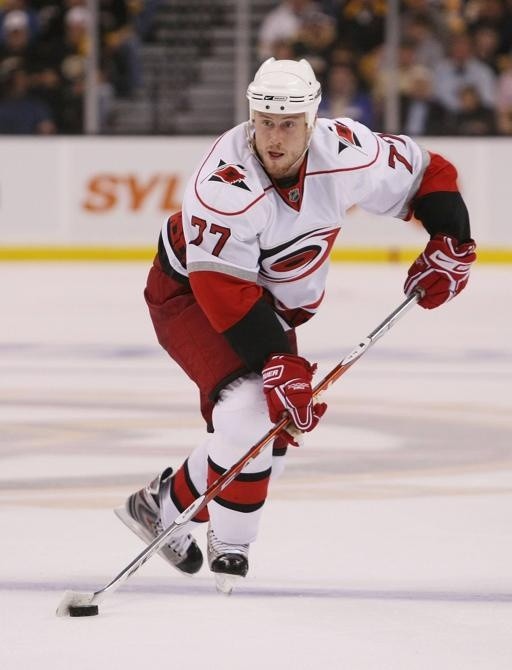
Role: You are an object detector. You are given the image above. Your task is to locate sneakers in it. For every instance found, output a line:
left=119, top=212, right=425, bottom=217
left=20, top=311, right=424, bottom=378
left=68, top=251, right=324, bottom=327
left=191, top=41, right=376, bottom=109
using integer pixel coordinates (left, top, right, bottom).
left=125, top=466, right=204, bottom=575
left=206, top=520, right=253, bottom=578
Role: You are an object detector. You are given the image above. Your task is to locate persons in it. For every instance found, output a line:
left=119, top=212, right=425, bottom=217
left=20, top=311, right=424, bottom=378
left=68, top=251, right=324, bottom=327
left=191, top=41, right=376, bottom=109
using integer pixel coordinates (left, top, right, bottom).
left=258, top=0, right=512, bottom=135
left=126, top=57, right=478, bottom=578
left=0, top=0, right=159, bottom=134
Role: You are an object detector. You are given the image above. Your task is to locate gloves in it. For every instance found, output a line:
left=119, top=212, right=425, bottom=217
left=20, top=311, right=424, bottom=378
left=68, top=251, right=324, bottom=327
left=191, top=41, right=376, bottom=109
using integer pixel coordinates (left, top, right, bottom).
left=261, top=351, right=329, bottom=437
left=403, top=232, right=478, bottom=309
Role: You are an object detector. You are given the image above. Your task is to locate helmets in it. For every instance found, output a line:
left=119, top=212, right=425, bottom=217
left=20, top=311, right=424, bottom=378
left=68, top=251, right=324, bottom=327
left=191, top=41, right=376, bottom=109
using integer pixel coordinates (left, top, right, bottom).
left=245, top=55, right=324, bottom=130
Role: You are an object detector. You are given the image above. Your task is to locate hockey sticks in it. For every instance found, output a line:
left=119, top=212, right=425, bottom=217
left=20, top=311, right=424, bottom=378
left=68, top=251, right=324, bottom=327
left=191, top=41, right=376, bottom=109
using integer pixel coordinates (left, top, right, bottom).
left=57, top=285, right=427, bottom=618
